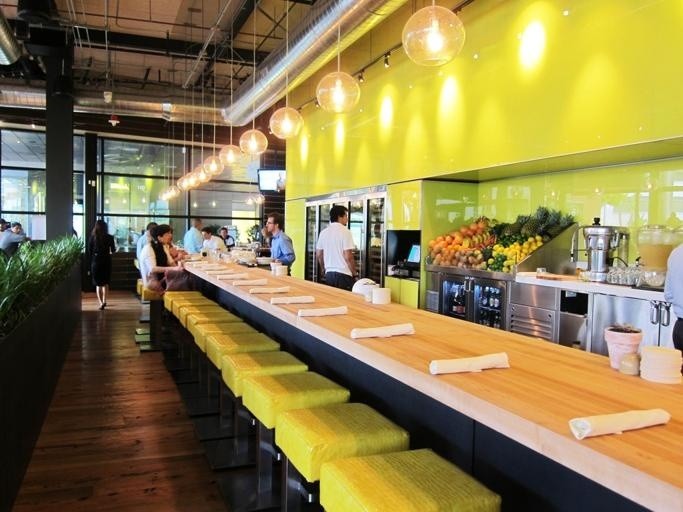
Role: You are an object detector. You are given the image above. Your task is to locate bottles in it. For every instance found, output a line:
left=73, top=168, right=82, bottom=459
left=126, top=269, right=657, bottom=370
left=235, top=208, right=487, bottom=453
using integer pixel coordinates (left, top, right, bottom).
left=210, top=248, right=232, bottom=263
left=445, top=281, right=502, bottom=327
left=636, top=225, right=683, bottom=272
left=621, top=354, right=640, bottom=375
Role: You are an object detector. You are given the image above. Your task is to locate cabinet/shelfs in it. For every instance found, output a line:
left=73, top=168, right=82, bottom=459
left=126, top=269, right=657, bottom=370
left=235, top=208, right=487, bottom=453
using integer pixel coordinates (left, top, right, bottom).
left=592, top=292, right=678, bottom=358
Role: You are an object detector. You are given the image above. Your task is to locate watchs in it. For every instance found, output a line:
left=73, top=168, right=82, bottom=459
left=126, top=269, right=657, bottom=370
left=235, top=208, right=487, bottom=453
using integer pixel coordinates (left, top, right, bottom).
left=353, top=272, right=359, bottom=278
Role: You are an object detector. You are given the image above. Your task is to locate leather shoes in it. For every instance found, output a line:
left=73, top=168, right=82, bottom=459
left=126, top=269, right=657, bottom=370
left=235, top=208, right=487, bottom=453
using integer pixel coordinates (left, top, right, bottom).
left=99, top=302, right=106, bottom=310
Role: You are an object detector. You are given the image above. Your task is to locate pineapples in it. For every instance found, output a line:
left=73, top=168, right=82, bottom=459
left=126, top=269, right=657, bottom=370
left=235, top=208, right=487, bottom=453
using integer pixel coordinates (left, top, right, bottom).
left=503, top=219, right=561, bottom=236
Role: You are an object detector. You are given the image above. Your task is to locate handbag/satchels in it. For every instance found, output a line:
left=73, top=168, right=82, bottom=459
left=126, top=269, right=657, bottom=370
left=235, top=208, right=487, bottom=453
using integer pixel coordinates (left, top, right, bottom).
left=145, top=270, right=192, bottom=291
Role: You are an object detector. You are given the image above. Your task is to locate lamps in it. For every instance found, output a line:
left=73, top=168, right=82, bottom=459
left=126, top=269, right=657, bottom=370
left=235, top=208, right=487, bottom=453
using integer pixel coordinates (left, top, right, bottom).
left=239, top=1, right=268, bottom=155
left=385, top=57, right=390, bottom=67
left=159, top=1, right=240, bottom=201
left=315, top=101, right=320, bottom=109
left=270, top=0, right=304, bottom=139
left=316, top=0, right=360, bottom=112
left=108, top=101, right=120, bottom=127
left=103, top=66, right=113, bottom=104
left=402, top=0, right=465, bottom=67
left=358, top=72, right=364, bottom=83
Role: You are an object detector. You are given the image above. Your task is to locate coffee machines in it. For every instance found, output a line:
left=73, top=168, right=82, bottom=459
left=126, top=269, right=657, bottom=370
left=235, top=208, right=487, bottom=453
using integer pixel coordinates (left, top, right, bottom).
left=582, top=216, right=629, bottom=280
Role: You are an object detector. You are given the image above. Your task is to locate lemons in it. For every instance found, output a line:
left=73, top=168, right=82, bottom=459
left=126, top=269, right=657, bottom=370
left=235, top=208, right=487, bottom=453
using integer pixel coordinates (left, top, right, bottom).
left=475, top=234, right=543, bottom=272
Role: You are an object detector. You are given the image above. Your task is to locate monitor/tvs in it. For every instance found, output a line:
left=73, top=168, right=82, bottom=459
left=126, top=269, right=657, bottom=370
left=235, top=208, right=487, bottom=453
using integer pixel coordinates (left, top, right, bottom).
left=403, top=241, right=421, bottom=268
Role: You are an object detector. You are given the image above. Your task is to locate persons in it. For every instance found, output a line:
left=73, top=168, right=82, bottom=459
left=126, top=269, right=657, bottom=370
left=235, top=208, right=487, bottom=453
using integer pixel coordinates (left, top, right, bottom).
left=277, top=172, right=285, bottom=190
left=0, top=219, right=31, bottom=258
left=201, top=227, right=228, bottom=254
left=664, top=242, right=683, bottom=375
left=262, top=226, right=273, bottom=250
left=266, top=212, right=295, bottom=276
left=183, top=217, right=205, bottom=253
left=316, top=205, right=359, bottom=293
left=72, top=220, right=116, bottom=310
left=219, top=227, right=235, bottom=252
left=127, top=222, right=191, bottom=295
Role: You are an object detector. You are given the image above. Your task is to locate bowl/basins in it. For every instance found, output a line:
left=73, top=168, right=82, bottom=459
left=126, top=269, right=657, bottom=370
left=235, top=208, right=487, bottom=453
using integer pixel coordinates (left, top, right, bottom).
left=256, top=257, right=274, bottom=264
left=644, top=271, right=665, bottom=287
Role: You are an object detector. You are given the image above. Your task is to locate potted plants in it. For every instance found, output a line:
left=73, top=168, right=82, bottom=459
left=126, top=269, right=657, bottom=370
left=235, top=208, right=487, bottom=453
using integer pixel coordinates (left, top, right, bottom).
left=604, top=323, right=643, bottom=369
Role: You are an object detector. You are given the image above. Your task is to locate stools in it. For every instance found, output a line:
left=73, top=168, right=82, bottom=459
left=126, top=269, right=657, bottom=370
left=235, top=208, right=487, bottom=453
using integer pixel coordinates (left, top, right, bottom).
left=213, top=372, right=350, bottom=511
left=135, top=279, right=162, bottom=351
left=319, top=448, right=502, bottom=512
left=250, top=402, right=410, bottom=512
left=164, top=291, right=280, bottom=441
left=204, top=350, right=307, bottom=469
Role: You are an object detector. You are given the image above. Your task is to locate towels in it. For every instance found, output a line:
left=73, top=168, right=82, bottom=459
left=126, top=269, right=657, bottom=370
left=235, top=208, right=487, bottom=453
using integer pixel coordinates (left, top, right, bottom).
left=430, top=352, right=510, bottom=375
left=186, top=260, right=233, bottom=275
left=567, top=408, right=671, bottom=442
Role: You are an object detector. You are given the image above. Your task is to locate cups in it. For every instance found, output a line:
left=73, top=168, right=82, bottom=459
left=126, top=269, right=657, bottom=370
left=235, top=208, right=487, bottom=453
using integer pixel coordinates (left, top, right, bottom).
left=609, top=267, right=642, bottom=287
left=363, top=282, right=392, bottom=305
left=271, top=262, right=288, bottom=277
left=536, top=267, right=546, bottom=275
